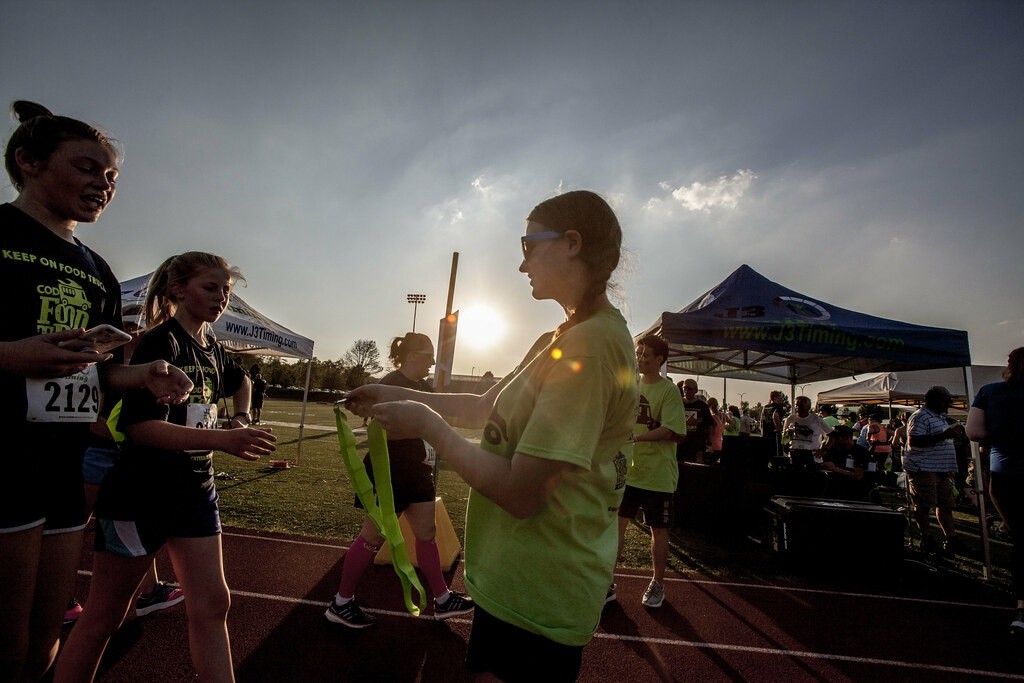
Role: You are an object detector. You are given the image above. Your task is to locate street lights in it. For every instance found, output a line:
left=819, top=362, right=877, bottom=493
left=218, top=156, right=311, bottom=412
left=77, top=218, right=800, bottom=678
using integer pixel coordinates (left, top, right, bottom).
left=407, top=294, right=426, bottom=333
left=797, top=384, right=811, bottom=396
left=737, top=393, right=746, bottom=410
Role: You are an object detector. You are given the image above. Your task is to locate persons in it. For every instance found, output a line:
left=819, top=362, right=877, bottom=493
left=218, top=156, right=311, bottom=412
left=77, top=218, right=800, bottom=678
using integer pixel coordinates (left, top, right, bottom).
left=51, top=251, right=277, bottom=683
left=604, top=334, right=686, bottom=608
left=1, top=98, right=194, bottom=682
left=965, top=346, right=1024, bottom=631
left=323, top=333, right=477, bottom=628
left=252, top=374, right=266, bottom=425
left=666, top=378, right=991, bottom=557
left=344, top=190, right=639, bottom=683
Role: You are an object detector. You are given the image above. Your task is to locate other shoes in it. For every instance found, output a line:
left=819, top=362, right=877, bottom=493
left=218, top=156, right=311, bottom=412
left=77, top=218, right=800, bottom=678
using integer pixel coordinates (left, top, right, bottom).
left=943, top=534, right=982, bottom=552
left=920, top=537, right=943, bottom=551
left=1008, top=608, right=1024, bottom=635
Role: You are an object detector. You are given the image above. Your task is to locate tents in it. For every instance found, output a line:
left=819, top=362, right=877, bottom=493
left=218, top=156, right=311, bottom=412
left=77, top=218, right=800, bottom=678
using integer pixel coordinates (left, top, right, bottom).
left=117, top=270, right=314, bottom=467
left=816, top=365, right=1007, bottom=420
left=631, top=264, right=994, bottom=582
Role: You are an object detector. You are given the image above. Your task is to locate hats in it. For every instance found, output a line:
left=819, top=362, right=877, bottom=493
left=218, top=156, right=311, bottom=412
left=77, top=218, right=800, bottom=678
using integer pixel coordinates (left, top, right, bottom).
left=682, top=379, right=697, bottom=388
left=827, top=426, right=853, bottom=436
left=123, top=315, right=147, bottom=330
left=707, top=398, right=718, bottom=405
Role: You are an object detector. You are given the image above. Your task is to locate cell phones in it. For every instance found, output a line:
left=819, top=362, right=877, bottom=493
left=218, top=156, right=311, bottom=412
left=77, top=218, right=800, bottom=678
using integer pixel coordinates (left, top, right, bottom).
left=57, top=324, right=132, bottom=355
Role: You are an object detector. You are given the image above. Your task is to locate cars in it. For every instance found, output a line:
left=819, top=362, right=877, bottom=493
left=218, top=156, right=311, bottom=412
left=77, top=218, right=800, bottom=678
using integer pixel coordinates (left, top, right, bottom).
left=267, top=384, right=351, bottom=394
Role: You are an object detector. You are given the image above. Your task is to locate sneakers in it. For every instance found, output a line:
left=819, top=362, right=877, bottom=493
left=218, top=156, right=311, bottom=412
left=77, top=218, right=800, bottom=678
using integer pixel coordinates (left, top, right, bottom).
left=136, top=582, right=185, bottom=616
left=325, top=598, right=375, bottom=628
left=434, top=591, right=474, bottom=618
left=605, top=582, right=616, bottom=601
left=61, top=602, right=84, bottom=631
left=642, top=581, right=665, bottom=607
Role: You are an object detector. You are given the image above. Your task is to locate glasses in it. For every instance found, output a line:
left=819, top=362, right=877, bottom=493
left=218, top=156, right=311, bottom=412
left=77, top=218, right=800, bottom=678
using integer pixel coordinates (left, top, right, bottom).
left=408, top=351, right=435, bottom=360
left=520, top=231, right=587, bottom=261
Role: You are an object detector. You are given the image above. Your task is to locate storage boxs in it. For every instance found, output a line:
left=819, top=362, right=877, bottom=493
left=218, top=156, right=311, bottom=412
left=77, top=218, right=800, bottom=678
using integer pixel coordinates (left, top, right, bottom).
left=766, top=495, right=905, bottom=587
